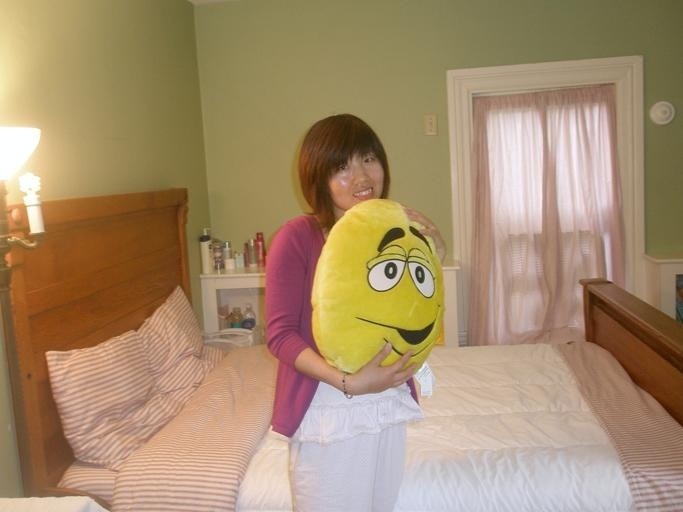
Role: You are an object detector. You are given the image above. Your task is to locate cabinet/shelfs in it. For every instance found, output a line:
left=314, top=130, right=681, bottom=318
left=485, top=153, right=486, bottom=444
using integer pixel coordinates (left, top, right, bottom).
left=200, top=252, right=460, bottom=345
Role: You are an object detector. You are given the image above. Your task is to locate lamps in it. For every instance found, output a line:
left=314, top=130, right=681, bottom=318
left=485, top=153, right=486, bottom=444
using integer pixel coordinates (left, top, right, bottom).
left=0, top=126, right=46, bottom=270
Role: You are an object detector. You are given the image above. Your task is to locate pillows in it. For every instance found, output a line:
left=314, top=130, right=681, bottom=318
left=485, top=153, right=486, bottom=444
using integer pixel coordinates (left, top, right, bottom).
left=40, top=284, right=226, bottom=470
left=311, top=196, right=446, bottom=379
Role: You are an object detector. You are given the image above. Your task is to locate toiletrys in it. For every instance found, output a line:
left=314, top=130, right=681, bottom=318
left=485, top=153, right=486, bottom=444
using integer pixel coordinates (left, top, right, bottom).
left=198, top=227, right=266, bottom=273
left=198, top=227, right=214, bottom=274
left=217, top=303, right=255, bottom=329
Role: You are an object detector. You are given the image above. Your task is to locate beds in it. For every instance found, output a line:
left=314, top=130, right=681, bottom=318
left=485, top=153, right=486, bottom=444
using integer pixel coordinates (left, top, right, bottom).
left=4, top=272, right=683, bottom=511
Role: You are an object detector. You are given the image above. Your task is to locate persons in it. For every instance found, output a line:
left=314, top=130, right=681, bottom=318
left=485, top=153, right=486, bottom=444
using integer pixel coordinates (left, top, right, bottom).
left=260, top=113, right=447, bottom=512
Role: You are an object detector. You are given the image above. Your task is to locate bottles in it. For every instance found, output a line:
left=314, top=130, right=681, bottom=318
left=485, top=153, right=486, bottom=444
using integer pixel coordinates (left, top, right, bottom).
left=199, top=228, right=265, bottom=275
left=218, top=304, right=255, bottom=333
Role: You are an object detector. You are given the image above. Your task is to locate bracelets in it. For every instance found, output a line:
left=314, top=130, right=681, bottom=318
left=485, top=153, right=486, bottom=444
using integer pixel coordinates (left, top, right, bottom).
left=342, top=371, right=352, bottom=399
